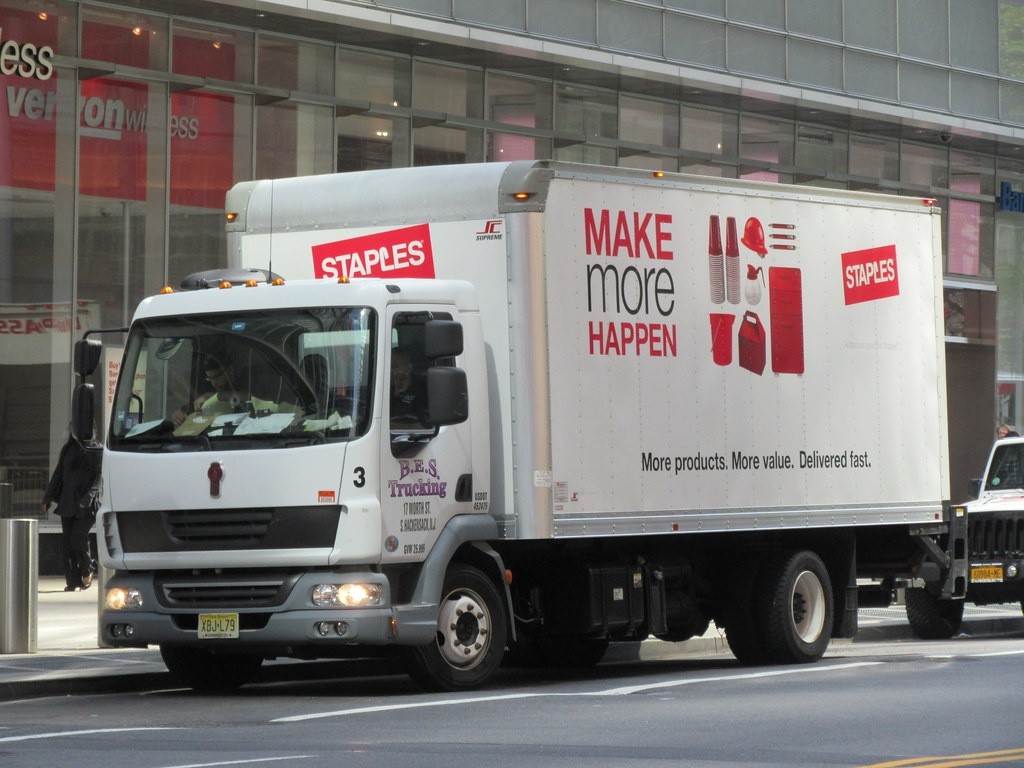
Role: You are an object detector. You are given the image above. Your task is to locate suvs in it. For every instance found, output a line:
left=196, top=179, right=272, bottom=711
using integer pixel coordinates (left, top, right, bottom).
left=903, top=435, right=1024, bottom=643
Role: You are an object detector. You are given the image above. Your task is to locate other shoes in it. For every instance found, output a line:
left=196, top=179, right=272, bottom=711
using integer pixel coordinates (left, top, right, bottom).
left=65, top=586, right=75, bottom=591
left=81, top=572, right=93, bottom=590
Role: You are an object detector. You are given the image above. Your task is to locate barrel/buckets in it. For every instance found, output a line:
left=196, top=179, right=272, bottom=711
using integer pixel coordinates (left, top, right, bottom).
left=710, top=313, right=734, bottom=365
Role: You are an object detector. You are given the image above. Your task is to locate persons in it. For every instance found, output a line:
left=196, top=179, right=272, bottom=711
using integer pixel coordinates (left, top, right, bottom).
left=42, top=417, right=106, bottom=592
left=173, top=352, right=252, bottom=424
left=356, top=346, right=430, bottom=436
left=987, top=424, right=1021, bottom=487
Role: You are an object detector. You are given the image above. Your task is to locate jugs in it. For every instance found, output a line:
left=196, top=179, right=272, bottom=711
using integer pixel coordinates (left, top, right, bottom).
left=744, top=265, right=766, bottom=305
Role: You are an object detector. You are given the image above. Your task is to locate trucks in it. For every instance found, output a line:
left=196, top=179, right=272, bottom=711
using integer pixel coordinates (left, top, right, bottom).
left=71, top=157, right=969, bottom=694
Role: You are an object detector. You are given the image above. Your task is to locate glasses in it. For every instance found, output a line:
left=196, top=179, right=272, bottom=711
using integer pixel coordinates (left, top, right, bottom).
left=205, top=372, right=221, bottom=381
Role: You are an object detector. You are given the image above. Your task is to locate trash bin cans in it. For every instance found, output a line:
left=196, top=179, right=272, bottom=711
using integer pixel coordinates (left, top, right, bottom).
left=0, top=518, right=40, bottom=656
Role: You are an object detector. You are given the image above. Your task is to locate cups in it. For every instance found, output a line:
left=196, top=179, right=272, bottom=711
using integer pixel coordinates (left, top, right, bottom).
left=708, top=216, right=741, bottom=305
